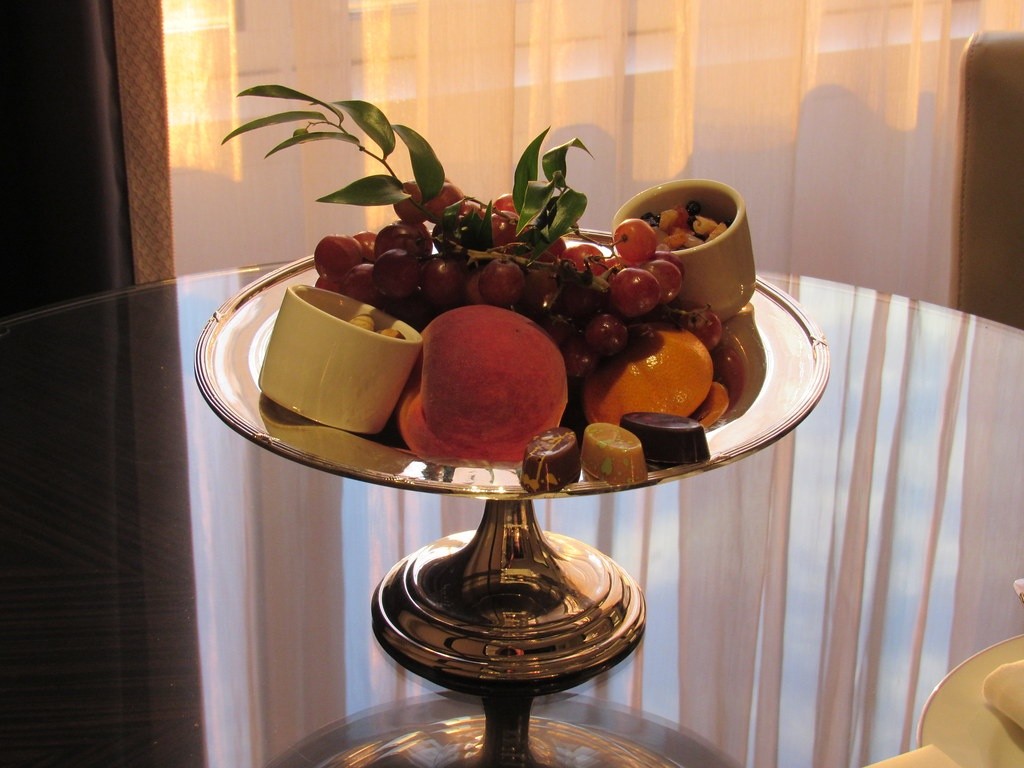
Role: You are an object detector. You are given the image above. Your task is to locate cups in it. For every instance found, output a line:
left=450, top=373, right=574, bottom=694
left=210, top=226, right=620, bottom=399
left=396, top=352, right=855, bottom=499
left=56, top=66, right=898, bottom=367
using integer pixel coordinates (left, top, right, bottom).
left=258, top=284, right=423, bottom=434
left=610, top=178, right=757, bottom=319
left=698, top=297, right=767, bottom=433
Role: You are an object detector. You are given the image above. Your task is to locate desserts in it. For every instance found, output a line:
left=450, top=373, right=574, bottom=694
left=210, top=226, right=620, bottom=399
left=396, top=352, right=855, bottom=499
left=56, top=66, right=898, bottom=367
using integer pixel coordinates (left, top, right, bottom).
left=520, top=411, right=711, bottom=494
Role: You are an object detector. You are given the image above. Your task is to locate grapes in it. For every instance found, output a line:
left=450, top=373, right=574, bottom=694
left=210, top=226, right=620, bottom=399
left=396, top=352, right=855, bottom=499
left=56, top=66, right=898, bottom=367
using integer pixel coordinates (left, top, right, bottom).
left=314, top=175, right=724, bottom=381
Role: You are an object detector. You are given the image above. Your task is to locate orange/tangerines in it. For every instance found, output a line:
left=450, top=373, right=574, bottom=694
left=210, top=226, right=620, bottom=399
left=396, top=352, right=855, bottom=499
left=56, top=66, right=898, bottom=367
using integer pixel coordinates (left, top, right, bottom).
left=582, top=320, right=712, bottom=427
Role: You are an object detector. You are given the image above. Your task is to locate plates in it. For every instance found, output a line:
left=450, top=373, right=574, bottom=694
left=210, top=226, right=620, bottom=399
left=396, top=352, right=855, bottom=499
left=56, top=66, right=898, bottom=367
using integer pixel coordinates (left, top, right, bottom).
left=916, top=633, right=1024, bottom=768
left=257, top=394, right=416, bottom=475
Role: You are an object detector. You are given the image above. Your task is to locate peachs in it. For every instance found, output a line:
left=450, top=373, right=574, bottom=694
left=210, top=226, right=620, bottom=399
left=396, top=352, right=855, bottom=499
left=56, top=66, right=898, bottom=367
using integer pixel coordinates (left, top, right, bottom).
left=395, top=305, right=566, bottom=461
